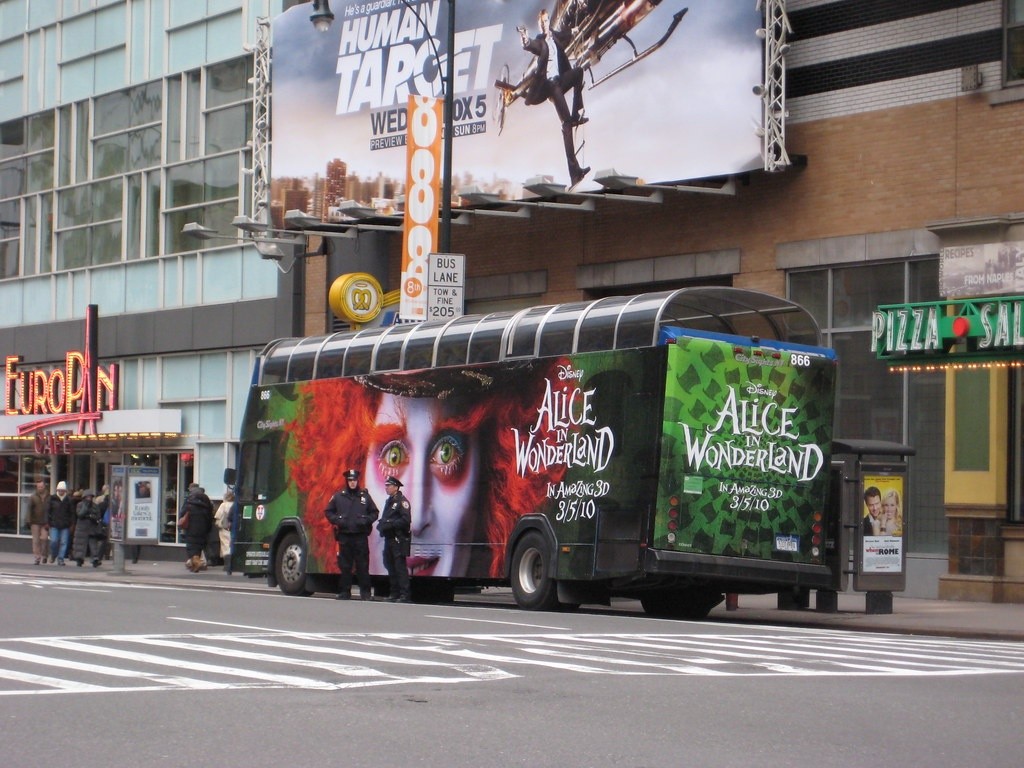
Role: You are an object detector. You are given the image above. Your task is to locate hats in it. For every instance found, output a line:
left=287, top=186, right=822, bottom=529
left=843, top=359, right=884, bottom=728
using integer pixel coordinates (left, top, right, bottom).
left=56, top=481, right=68, bottom=492
left=81, top=489, right=95, bottom=498
left=385, top=476, right=404, bottom=486
left=343, top=470, right=360, bottom=479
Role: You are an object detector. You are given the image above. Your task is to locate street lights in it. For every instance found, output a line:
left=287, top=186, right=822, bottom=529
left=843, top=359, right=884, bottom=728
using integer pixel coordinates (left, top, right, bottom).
left=309, top=0, right=456, bottom=253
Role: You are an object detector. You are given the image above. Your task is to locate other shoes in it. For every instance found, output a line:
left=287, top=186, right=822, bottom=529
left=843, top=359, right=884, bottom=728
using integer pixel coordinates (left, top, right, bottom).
left=383, top=595, right=397, bottom=602
left=338, top=589, right=351, bottom=599
left=50, top=559, right=54, bottom=563
left=77, top=561, right=82, bottom=566
left=361, top=590, right=371, bottom=600
left=42, top=559, right=46, bottom=563
left=400, top=595, right=408, bottom=602
left=58, top=561, right=66, bottom=566
left=34, top=560, right=39, bottom=565
left=132, top=558, right=137, bottom=564
left=93, top=562, right=102, bottom=567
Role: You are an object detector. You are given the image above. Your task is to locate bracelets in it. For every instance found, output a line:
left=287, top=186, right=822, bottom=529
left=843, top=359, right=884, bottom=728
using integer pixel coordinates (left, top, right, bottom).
left=880, top=527, right=886, bottom=532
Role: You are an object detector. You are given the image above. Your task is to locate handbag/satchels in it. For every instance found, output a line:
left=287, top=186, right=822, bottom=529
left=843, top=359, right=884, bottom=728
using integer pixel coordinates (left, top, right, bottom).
left=178, top=497, right=190, bottom=528
left=95, top=519, right=109, bottom=537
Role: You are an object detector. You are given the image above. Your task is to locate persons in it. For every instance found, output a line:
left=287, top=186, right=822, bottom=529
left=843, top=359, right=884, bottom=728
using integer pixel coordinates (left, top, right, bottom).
left=376, top=474, right=411, bottom=603
left=284, top=357, right=582, bottom=577
left=111, top=480, right=123, bottom=517
left=25, top=481, right=111, bottom=568
left=864, top=486, right=901, bottom=536
left=178, top=483, right=234, bottom=573
left=323, top=470, right=380, bottom=601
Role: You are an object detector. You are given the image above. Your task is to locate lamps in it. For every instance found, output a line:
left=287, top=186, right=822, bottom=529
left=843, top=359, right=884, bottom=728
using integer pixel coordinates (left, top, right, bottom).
left=779, top=44, right=791, bottom=57
left=756, top=28, right=766, bottom=40
left=180, top=168, right=737, bottom=275
left=752, top=83, right=765, bottom=96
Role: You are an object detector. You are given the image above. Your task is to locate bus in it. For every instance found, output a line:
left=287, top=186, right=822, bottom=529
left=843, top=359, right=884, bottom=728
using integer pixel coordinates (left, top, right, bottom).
left=223, top=287, right=834, bottom=621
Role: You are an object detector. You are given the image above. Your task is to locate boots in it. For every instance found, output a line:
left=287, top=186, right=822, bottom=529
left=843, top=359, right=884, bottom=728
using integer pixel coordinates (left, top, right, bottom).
left=191, top=555, right=203, bottom=573
